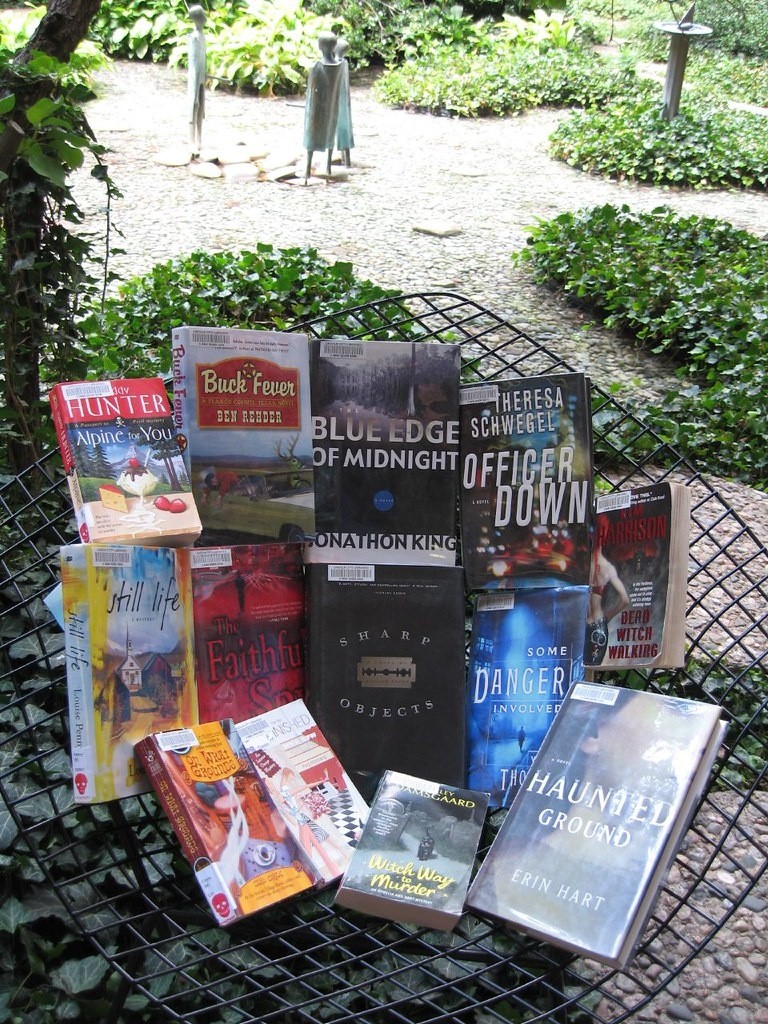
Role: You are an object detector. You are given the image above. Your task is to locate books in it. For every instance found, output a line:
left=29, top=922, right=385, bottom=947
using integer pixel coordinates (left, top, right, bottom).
left=47, top=323, right=731, bottom=974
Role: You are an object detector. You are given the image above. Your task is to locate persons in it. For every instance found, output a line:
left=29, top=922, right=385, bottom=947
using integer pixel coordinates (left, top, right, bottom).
left=186, top=3, right=209, bottom=165
left=302, top=31, right=356, bottom=188
